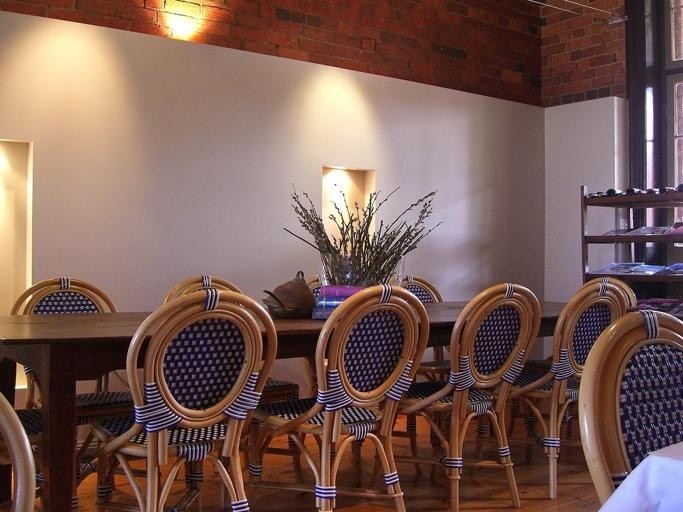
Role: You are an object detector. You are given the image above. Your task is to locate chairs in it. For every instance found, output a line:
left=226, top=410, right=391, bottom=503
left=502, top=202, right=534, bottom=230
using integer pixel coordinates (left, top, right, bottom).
left=475, top=276, right=642, bottom=504
left=308, top=275, right=428, bottom=450
left=0, top=388, right=41, bottom=512
left=7, top=275, right=181, bottom=489
left=243, top=283, right=431, bottom=511
left=577, top=310, right=683, bottom=511
left=390, top=276, right=452, bottom=446
left=88, top=287, right=280, bottom=511
left=366, top=280, right=545, bottom=509
left=163, top=277, right=302, bottom=491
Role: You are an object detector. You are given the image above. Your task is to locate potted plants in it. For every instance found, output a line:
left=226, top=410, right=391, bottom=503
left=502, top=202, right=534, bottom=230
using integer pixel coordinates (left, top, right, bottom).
left=282, top=181, right=445, bottom=321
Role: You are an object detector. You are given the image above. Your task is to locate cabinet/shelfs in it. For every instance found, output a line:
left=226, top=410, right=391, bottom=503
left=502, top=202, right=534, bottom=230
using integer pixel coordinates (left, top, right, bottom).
left=581, top=186, right=683, bottom=285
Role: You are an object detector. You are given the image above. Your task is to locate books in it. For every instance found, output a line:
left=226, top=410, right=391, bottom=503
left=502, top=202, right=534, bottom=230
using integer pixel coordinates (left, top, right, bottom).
left=319, top=284, right=366, bottom=298
left=627, top=225, right=671, bottom=236
left=654, top=261, right=682, bottom=277
left=634, top=297, right=679, bottom=314
left=311, top=307, right=337, bottom=321
left=668, top=302, right=682, bottom=318
left=622, top=263, right=666, bottom=277
left=666, top=220, right=682, bottom=235
left=316, top=296, right=348, bottom=308
left=598, top=228, right=637, bottom=237
left=587, top=261, right=644, bottom=276
